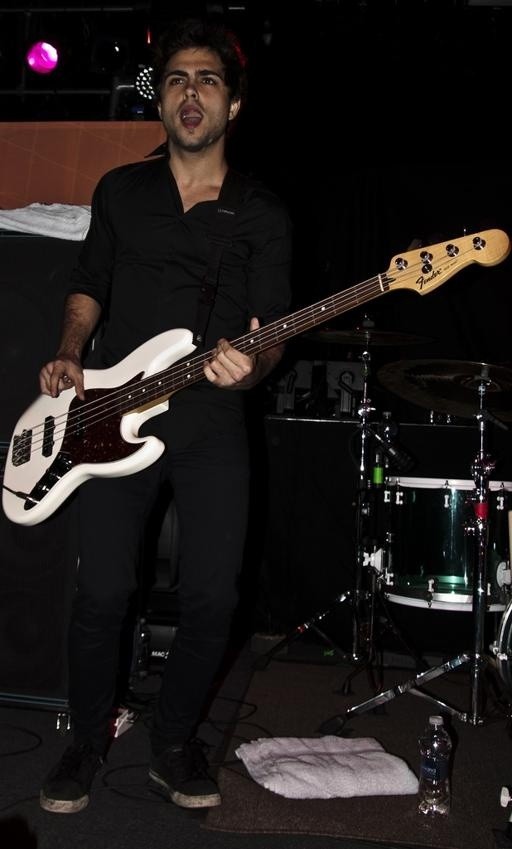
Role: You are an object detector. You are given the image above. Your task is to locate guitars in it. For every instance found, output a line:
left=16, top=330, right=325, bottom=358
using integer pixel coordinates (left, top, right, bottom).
left=3, top=228, right=509, bottom=525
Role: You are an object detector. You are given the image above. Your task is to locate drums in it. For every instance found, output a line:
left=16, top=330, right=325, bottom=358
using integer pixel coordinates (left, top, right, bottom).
left=379, top=476, right=512, bottom=613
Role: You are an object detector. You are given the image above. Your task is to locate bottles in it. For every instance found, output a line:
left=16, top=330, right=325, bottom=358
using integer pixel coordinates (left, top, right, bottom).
left=414, top=715, right=454, bottom=820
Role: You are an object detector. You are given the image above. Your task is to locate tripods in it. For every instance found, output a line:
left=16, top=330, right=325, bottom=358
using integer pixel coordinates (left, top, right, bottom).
left=253, top=344, right=429, bottom=674
left=320, top=379, right=512, bottom=736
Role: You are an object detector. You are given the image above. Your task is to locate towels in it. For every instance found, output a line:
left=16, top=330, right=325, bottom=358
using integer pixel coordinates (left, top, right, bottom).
left=0, top=200, right=92, bottom=243
left=235, top=733, right=423, bottom=799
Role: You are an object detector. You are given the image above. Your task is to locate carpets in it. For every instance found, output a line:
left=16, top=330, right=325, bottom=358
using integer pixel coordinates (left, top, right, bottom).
left=200, top=659, right=512, bottom=849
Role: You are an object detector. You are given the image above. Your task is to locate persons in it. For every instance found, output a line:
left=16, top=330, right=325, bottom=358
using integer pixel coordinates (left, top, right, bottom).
left=37, top=25, right=298, bottom=819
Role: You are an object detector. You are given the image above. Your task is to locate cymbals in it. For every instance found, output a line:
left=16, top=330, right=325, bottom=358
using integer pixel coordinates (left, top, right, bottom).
left=376, top=358, right=512, bottom=423
left=298, top=331, right=442, bottom=346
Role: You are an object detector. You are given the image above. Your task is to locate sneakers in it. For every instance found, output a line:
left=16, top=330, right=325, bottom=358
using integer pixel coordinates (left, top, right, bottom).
left=145, top=741, right=223, bottom=811
left=38, top=735, right=114, bottom=813
